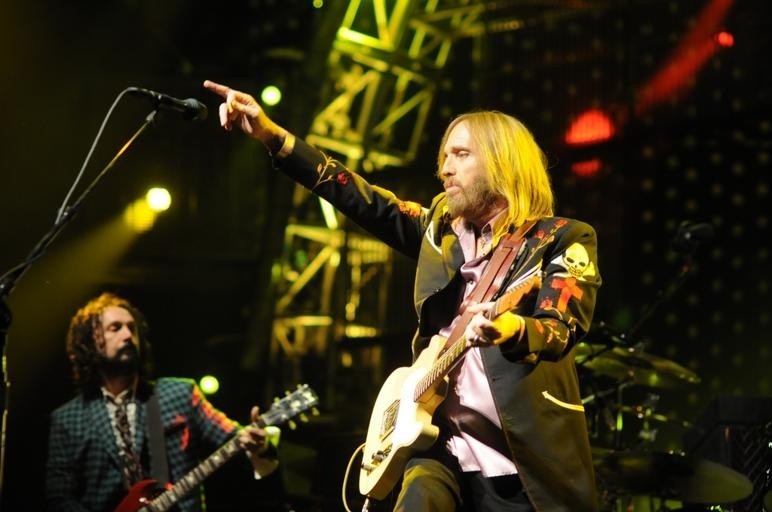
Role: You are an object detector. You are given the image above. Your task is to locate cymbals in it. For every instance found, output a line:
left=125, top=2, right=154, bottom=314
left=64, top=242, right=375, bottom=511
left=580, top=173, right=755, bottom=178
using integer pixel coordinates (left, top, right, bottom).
left=573, top=343, right=701, bottom=391
left=594, top=450, right=754, bottom=503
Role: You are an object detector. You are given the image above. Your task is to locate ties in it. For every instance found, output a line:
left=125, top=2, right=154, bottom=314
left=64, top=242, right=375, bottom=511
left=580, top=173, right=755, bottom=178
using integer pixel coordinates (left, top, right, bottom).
left=105, top=391, right=144, bottom=494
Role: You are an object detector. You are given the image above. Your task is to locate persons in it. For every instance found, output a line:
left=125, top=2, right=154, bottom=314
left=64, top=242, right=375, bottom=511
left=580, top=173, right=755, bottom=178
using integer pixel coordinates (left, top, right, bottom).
left=44, top=290, right=285, bottom=510
left=199, top=59, right=610, bottom=510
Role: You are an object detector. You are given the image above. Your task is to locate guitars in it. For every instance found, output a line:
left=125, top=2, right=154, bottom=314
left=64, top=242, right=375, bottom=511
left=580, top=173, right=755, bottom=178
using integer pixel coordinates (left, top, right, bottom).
left=359, top=277, right=540, bottom=500
left=114, top=382, right=320, bottom=511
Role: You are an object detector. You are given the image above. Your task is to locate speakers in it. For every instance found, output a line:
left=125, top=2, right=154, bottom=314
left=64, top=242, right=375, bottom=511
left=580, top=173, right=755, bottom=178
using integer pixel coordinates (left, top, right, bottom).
left=700, top=396, right=772, bottom=512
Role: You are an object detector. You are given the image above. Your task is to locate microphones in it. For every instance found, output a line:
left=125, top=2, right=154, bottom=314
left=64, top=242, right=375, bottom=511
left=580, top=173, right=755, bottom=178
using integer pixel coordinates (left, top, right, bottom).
left=132, top=85, right=209, bottom=120
